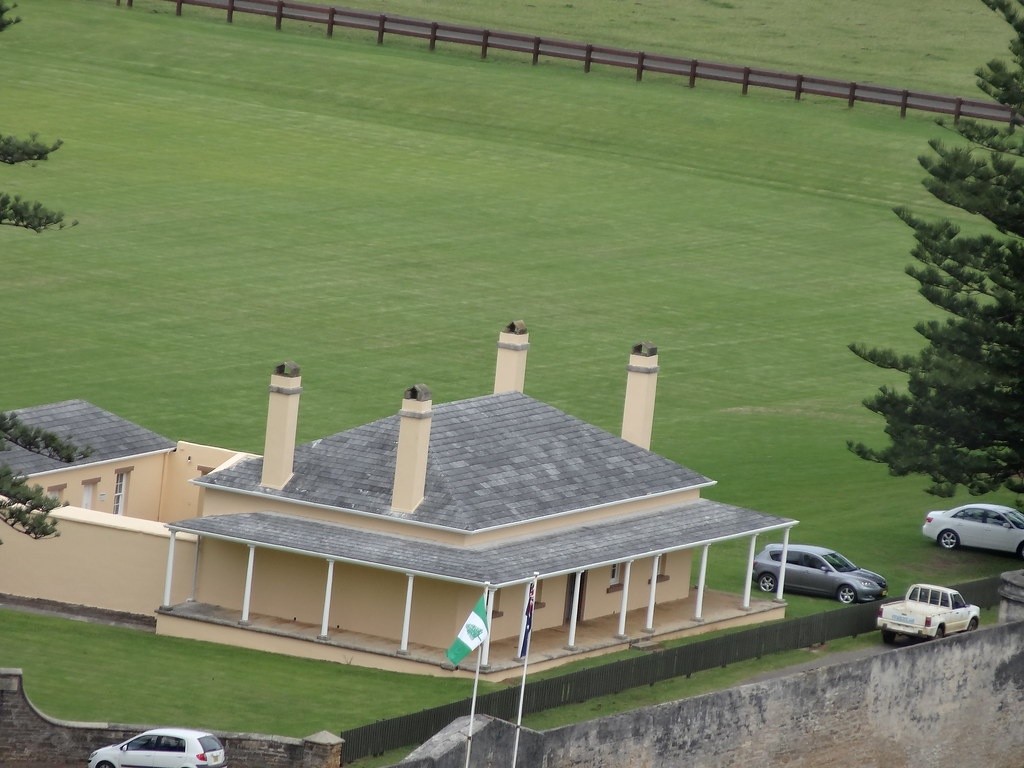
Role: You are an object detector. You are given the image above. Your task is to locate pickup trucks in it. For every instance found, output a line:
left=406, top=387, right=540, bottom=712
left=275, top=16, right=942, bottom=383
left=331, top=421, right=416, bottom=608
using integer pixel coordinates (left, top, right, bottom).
left=876, top=584, right=981, bottom=646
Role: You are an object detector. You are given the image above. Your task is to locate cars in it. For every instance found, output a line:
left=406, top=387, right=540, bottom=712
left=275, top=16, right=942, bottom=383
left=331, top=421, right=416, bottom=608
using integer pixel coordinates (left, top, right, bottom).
left=922, top=503, right=1024, bottom=563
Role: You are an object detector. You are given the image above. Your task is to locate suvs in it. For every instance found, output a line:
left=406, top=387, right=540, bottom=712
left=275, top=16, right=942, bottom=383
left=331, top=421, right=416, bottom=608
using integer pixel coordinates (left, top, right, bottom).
left=751, top=544, right=889, bottom=604
left=87, top=727, right=228, bottom=768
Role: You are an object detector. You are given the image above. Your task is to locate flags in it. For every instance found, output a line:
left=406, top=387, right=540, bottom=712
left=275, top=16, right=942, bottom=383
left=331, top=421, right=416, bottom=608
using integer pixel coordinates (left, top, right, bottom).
left=519, top=584, right=533, bottom=658
left=446, top=596, right=489, bottom=669
